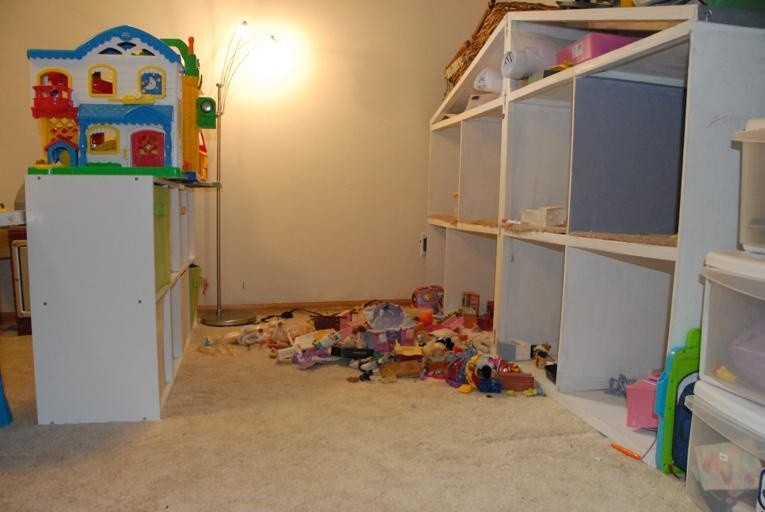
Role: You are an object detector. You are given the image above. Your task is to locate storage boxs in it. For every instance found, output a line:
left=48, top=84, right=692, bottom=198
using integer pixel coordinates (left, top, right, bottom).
left=683, top=380, right=765, bottom=512
left=695, top=247, right=765, bottom=410
left=730, top=115, right=765, bottom=256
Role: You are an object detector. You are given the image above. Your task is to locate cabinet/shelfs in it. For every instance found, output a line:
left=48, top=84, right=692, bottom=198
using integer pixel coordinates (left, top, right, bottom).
left=427, top=5, right=765, bottom=470
left=24, top=173, right=199, bottom=425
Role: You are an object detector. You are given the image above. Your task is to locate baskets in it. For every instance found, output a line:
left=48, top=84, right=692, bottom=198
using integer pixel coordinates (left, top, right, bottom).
left=446, top=2, right=567, bottom=88
